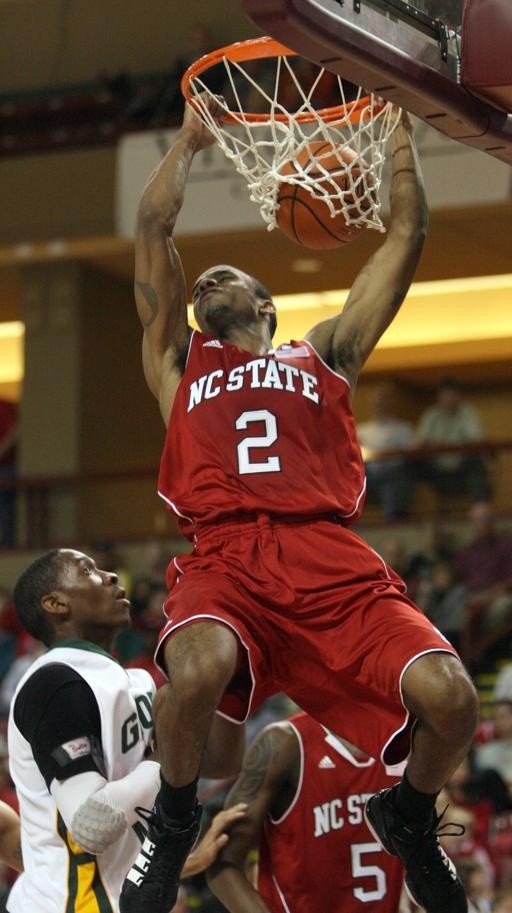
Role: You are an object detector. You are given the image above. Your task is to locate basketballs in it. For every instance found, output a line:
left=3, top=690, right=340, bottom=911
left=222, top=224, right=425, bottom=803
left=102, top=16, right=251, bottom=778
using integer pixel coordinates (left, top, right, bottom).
left=275, top=142, right=377, bottom=249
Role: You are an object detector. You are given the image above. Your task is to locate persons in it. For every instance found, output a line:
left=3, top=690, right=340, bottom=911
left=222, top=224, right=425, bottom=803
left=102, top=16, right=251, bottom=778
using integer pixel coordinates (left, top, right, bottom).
left=118, top=89, right=486, bottom=912
left=354, top=376, right=511, bottom=526
left=0, top=504, right=512, bottom=913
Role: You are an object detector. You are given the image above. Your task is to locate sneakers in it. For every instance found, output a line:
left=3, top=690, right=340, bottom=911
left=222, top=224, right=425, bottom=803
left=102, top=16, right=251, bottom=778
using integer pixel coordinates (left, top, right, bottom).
left=117, top=792, right=204, bottom=912
left=363, top=782, right=471, bottom=912
